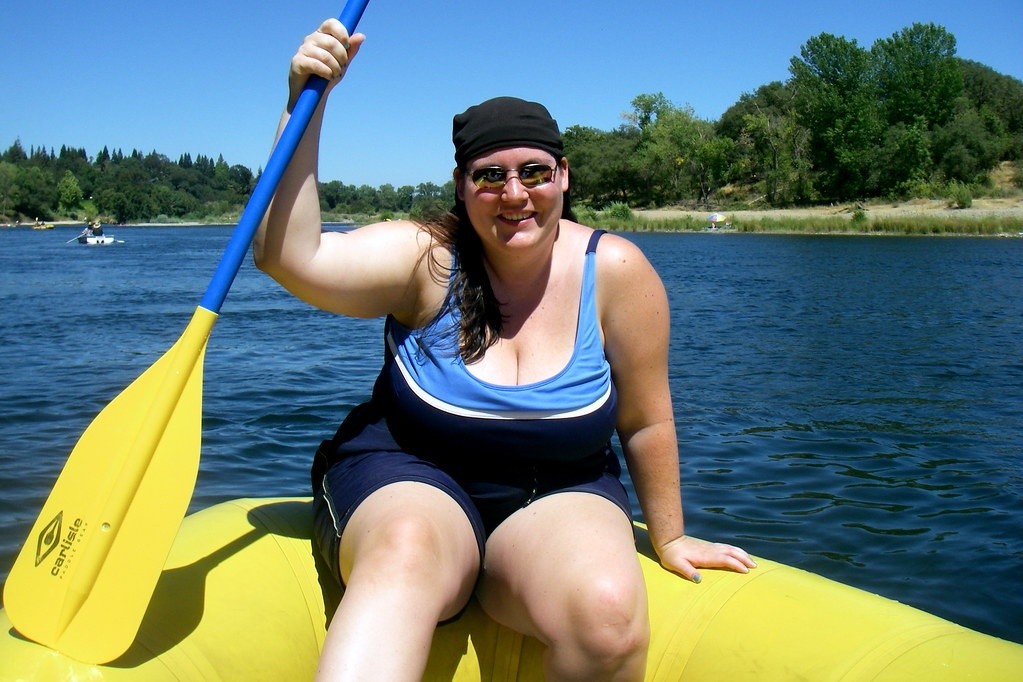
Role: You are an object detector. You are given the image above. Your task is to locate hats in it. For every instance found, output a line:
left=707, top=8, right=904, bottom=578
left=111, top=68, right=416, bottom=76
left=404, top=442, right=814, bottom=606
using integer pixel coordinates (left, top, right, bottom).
left=454, top=96, right=564, bottom=167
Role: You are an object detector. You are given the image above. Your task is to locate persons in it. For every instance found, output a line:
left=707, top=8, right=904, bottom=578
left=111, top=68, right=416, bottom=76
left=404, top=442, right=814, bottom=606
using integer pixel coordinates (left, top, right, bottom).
left=251, top=17, right=756, bottom=682
left=41, top=220, right=45, bottom=225
left=712, top=222, right=717, bottom=228
left=82, top=222, right=103, bottom=236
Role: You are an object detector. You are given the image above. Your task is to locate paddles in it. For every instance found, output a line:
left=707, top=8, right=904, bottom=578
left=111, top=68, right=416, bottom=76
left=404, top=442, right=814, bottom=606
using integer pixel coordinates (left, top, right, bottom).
left=35, top=216, right=39, bottom=221
left=0, top=0, right=368, bottom=664
left=67, top=233, right=84, bottom=244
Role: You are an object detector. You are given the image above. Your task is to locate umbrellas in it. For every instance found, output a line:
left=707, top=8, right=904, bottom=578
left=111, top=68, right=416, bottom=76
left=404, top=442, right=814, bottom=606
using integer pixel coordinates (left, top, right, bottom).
left=707, top=213, right=726, bottom=222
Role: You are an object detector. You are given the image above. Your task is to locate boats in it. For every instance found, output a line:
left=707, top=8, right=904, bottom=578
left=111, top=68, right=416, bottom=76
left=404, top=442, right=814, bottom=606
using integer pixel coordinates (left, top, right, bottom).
left=32, top=217, right=55, bottom=230
left=77, top=235, right=115, bottom=246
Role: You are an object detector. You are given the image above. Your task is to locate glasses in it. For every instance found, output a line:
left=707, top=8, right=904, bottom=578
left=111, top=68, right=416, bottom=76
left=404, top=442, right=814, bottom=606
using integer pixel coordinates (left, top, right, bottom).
left=465, top=161, right=559, bottom=190
